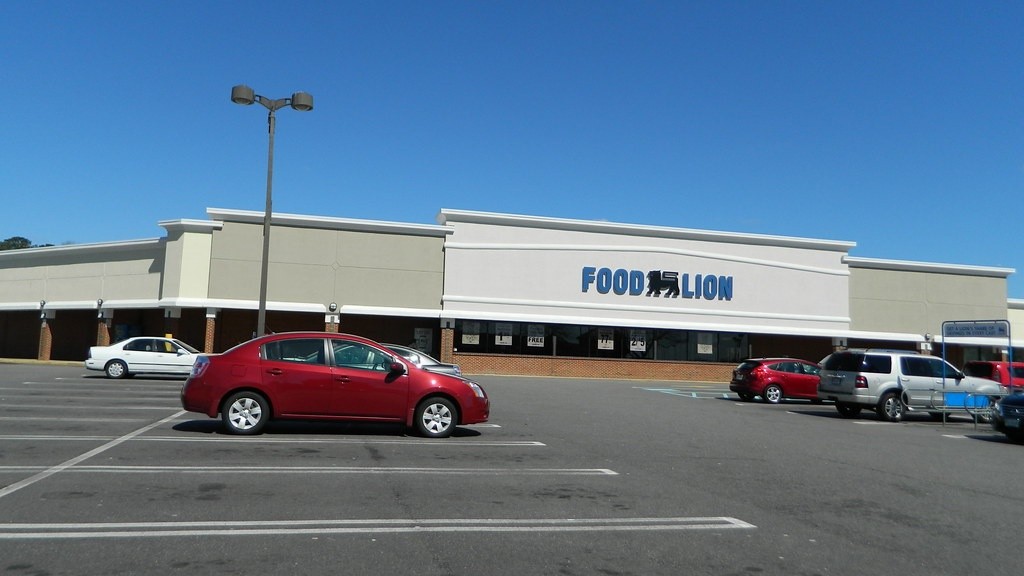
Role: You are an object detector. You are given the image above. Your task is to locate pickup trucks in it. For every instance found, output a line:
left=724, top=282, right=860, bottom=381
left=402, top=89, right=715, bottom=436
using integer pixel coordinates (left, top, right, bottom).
left=818, top=349, right=1010, bottom=422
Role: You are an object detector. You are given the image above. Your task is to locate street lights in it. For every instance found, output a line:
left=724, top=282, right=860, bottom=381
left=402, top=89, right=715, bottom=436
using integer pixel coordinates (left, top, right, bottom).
left=231, top=86, right=315, bottom=335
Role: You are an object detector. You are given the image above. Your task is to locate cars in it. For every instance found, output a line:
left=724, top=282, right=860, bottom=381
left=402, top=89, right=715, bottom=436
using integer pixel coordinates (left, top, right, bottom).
left=180, top=331, right=490, bottom=438
left=85, top=336, right=213, bottom=380
left=991, top=392, right=1024, bottom=443
left=729, top=357, right=822, bottom=404
left=962, top=360, right=1024, bottom=387
left=284, top=342, right=462, bottom=378
